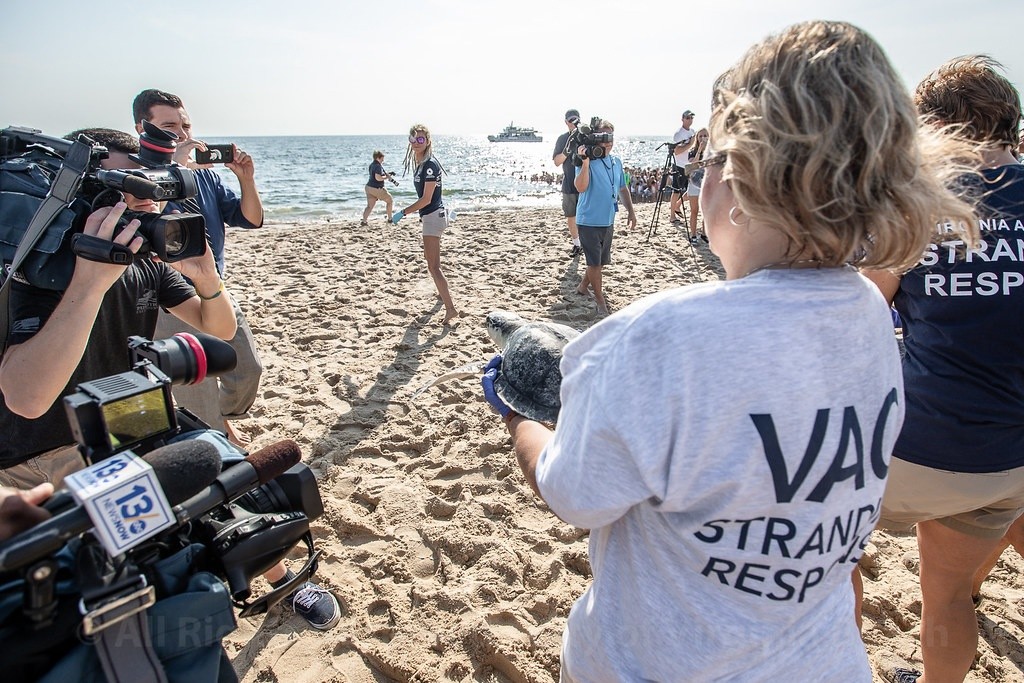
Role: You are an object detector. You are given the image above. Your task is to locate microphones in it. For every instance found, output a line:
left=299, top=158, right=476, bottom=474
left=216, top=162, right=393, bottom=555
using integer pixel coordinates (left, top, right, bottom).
left=95, top=170, right=164, bottom=200
left=0, top=439, right=302, bottom=576
left=578, top=124, right=590, bottom=135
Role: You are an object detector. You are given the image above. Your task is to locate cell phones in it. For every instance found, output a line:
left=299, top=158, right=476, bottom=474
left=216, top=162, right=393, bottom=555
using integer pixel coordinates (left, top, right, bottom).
left=197, top=144, right=234, bottom=164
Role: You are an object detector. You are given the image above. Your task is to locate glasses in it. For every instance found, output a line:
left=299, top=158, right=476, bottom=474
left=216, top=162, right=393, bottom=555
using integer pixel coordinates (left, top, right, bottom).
left=684, top=155, right=727, bottom=189
left=409, top=136, right=428, bottom=144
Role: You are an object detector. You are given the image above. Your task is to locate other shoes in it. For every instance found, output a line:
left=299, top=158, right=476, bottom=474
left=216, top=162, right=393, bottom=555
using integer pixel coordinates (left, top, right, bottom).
left=569, top=245, right=584, bottom=257
left=361, top=222, right=370, bottom=226
left=388, top=220, right=393, bottom=223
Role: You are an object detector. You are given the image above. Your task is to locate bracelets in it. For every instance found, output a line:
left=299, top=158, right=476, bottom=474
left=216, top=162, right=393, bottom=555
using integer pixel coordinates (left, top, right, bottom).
left=195, top=278, right=224, bottom=300
left=403, top=209, right=406, bottom=216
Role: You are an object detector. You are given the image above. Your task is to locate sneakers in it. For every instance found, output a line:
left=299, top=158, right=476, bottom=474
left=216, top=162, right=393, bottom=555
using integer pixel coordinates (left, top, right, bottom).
left=284, top=582, right=341, bottom=630
left=701, top=235, right=709, bottom=243
left=692, top=238, right=698, bottom=245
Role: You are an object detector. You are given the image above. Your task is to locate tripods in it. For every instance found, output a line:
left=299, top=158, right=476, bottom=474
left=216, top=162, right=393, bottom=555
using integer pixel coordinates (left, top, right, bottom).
left=645, top=142, right=693, bottom=244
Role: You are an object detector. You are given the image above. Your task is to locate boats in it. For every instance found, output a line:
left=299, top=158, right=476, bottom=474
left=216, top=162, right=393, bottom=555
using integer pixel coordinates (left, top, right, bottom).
left=487, top=120, right=542, bottom=142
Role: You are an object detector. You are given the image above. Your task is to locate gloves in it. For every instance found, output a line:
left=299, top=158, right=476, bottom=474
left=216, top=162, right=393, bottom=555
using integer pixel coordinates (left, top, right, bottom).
left=481, top=355, right=518, bottom=421
left=392, top=211, right=404, bottom=225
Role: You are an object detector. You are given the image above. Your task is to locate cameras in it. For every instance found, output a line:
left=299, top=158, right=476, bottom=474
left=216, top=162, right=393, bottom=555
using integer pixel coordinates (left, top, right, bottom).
left=389, top=179, right=399, bottom=186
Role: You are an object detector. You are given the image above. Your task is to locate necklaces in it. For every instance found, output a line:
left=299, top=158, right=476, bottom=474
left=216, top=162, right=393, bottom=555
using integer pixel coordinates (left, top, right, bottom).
left=743, top=256, right=834, bottom=279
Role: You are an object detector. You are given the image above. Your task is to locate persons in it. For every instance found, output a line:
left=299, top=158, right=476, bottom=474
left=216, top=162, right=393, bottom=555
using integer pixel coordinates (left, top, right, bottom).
left=531, top=170, right=564, bottom=185
left=361, top=150, right=393, bottom=226
left=623, top=166, right=673, bottom=204
left=391, top=124, right=459, bottom=325
left=670, top=110, right=709, bottom=245
left=849, top=56, right=1024, bottom=683
left=0, top=89, right=340, bottom=683
left=481, top=20, right=981, bottom=683
left=552, top=109, right=637, bottom=315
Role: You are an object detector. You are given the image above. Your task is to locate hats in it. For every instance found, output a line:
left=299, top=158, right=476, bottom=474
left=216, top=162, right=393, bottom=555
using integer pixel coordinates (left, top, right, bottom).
left=566, top=109, right=580, bottom=121
left=683, top=111, right=695, bottom=116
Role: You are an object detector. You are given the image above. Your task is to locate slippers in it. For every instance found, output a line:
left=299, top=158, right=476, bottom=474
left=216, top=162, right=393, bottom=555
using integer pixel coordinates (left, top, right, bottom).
left=670, top=220, right=683, bottom=225
left=675, top=211, right=684, bottom=218
